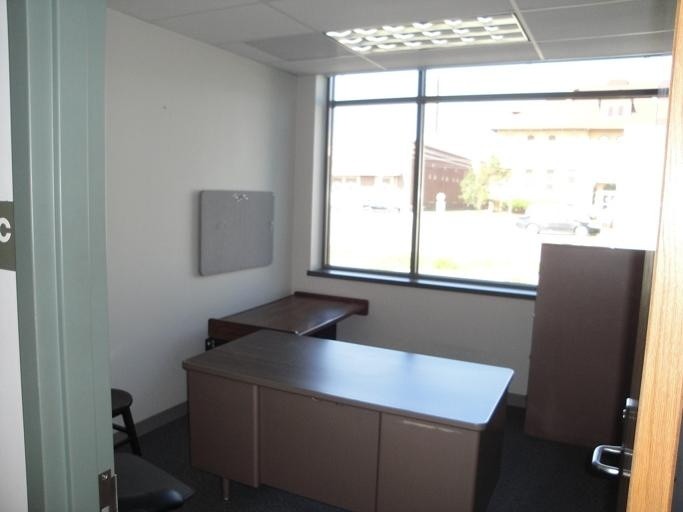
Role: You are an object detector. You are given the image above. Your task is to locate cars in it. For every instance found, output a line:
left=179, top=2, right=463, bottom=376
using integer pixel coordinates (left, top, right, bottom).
left=515, top=206, right=603, bottom=238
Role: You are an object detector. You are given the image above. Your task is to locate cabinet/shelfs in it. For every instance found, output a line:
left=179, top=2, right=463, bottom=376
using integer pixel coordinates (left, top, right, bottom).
left=522, top=242, right=646, bottom=448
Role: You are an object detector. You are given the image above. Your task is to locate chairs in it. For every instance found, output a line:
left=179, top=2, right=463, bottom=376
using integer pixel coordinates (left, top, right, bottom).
left=113, top=449, right=196, bottom=510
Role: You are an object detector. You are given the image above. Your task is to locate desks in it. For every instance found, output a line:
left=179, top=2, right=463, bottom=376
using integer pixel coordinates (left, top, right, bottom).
left=207, top=291, right=370, bottom=346
left=181, top=327, right=516, bottom=511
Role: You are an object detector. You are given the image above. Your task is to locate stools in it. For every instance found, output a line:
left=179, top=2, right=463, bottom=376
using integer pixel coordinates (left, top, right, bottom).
left=111, top=387, right=142, bottom=457
